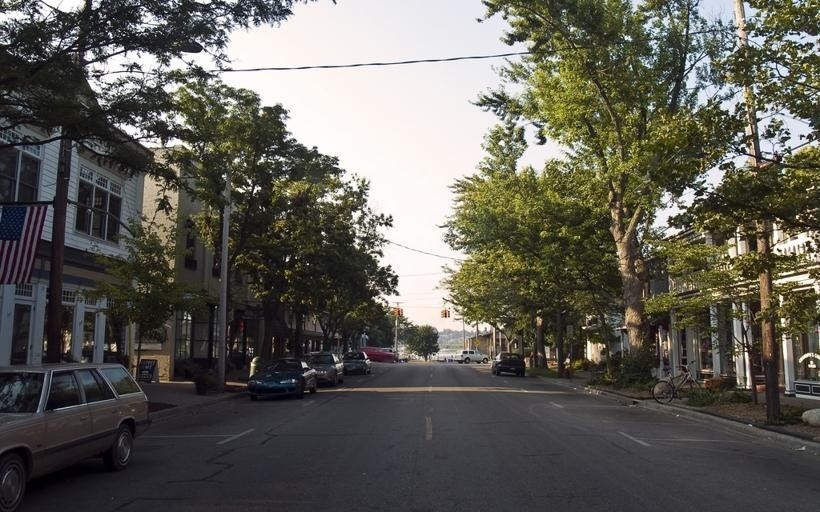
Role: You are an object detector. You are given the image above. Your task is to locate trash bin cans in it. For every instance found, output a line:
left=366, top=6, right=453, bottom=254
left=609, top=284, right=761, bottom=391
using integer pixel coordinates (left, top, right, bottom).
left=249, top=356, right=265, bottom=377
left=194, top=358, right=220, bottom=396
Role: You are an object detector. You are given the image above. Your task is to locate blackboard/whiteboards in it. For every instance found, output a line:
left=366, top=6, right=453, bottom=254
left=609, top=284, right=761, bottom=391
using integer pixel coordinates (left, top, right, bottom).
left=136, top=359, right=158, bottom=383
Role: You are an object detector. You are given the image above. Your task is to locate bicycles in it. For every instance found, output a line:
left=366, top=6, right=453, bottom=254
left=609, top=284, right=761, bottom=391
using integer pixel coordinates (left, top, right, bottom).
left=652, top=360, right=703, bottom=405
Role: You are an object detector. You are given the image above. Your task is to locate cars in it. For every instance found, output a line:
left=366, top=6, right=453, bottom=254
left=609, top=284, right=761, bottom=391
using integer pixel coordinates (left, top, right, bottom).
left=342, top=351, right=372, bottom=375
left=246, top=352, right=344, bottom=401
left=492, top=351, right=525, bottom=376
left=0, top=362, right=152, bottom=512
left=359, top=346, right=410, bottom=363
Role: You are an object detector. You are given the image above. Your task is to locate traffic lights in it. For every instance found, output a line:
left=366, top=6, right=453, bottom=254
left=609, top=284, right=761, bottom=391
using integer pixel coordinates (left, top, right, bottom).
left=394, top=307, right=404, bottom=318
left=441, top=309, right=450, bottom=319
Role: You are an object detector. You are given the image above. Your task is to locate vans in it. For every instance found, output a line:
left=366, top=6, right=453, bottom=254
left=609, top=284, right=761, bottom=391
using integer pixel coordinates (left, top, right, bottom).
left=455, top=349, right=490, bottom=364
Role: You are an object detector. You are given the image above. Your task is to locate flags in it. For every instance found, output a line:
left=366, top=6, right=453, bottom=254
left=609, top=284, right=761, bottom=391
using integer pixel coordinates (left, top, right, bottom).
left=0, top=203, right=48, bottom=285
